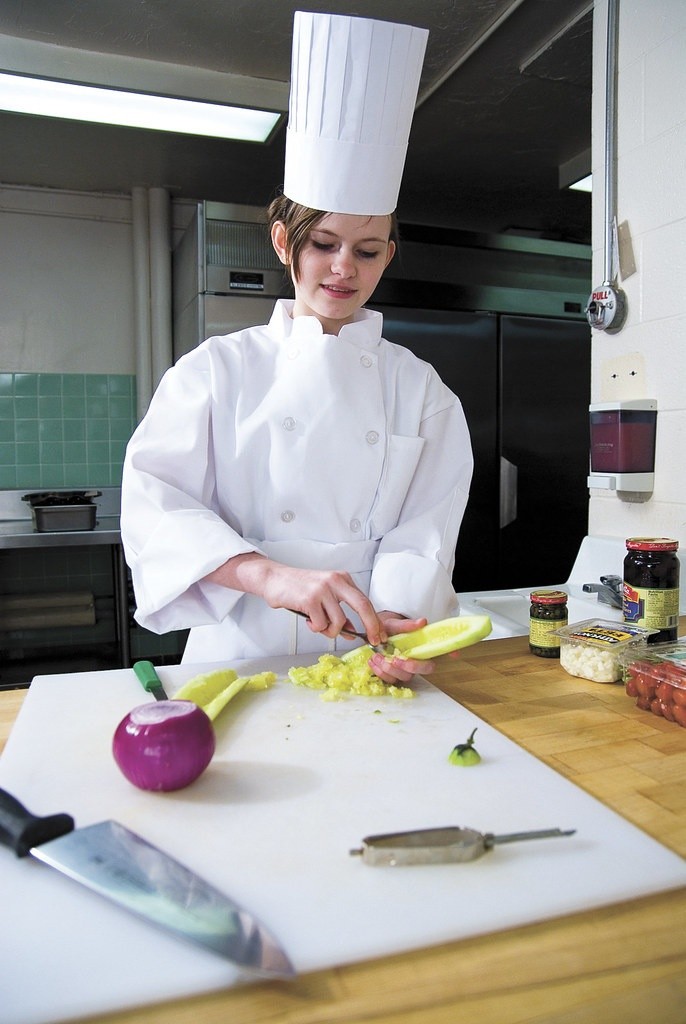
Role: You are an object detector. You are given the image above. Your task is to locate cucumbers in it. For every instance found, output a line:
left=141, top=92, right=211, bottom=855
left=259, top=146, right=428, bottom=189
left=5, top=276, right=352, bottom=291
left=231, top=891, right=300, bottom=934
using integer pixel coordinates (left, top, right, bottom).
left=342, top=613, right=492, bottom=661
left=171, top=670, right=249, bottom=722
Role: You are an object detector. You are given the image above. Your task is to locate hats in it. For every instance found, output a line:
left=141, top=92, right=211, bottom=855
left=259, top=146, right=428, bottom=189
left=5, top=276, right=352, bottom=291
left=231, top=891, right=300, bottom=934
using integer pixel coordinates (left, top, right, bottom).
left=282, top=11, right=429, bottom=216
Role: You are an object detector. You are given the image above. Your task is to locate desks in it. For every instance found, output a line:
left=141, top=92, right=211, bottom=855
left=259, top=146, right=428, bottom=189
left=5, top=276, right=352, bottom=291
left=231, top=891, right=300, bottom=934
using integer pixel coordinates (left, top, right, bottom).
left=0, top=631, right=686, bottom=1024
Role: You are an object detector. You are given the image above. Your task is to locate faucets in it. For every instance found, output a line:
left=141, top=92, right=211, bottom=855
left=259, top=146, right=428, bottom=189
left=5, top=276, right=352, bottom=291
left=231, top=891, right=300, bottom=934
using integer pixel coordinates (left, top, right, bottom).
left=582, top=573, right=623, bottom=610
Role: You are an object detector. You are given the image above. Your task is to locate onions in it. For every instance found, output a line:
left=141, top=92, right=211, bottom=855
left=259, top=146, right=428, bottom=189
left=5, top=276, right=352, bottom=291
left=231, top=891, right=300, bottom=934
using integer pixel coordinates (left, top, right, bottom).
left=111, top=700, right=215, bottom=792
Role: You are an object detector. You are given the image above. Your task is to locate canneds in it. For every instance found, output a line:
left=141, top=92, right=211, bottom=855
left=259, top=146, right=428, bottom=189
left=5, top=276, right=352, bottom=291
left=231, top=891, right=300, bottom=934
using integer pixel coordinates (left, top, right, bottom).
left=622, top=537, right=680, bottom=643
left=529, top=589, right=569, bottom=658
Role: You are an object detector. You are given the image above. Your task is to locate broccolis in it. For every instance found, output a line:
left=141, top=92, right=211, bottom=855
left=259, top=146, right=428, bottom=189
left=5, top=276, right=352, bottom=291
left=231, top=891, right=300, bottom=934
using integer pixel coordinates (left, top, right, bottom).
left=560, top=645, right=622, bottom=683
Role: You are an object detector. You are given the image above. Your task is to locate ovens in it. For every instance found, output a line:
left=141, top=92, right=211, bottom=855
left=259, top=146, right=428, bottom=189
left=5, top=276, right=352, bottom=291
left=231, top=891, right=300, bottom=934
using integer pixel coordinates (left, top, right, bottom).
left=0, top=543, right=121, bottom=691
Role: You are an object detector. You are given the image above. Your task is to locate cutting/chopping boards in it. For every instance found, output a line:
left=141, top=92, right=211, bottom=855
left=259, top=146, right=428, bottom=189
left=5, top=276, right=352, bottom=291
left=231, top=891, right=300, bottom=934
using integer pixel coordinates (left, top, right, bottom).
left=0, top=649, right=686, bottom=1024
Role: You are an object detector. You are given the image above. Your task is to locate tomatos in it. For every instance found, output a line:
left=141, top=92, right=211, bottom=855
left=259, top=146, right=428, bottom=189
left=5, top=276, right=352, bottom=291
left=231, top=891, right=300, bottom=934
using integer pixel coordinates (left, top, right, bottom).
left=622, top=655, right=686, bottom=727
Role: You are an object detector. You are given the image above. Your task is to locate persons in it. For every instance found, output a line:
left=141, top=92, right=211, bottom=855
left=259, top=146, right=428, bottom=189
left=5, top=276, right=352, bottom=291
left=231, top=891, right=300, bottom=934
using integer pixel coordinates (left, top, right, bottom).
left=120, top=190, right=474, bottom=685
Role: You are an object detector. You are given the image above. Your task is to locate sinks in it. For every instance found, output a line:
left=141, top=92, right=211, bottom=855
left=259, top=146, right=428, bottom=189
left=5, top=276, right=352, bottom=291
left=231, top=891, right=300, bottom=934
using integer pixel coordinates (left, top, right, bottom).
left=458, top=582, right=624, bottom=645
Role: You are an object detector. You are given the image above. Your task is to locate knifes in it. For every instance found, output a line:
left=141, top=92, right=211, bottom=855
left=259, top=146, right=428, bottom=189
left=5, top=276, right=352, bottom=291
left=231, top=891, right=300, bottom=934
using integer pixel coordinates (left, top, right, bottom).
left=0, top=785, right=298, bottom=981
left=133, top=661, right=172, bottom=702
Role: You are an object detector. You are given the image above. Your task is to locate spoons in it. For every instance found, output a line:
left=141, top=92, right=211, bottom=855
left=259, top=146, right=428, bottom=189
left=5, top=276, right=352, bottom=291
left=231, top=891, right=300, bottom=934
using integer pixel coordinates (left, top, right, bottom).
left=284, top=608, right=395, bottom=657
